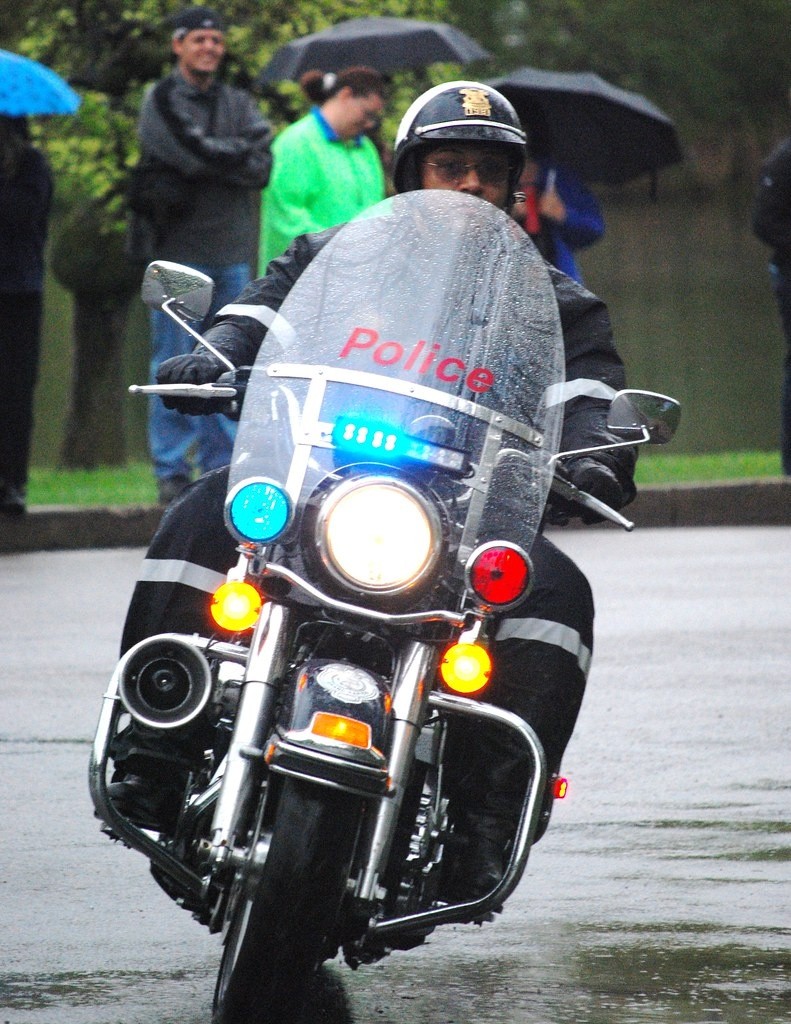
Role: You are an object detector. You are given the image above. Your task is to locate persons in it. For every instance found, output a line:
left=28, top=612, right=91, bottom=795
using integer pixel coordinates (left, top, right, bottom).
left=0, top=113, right=56, bottom=518
left=255, top=67, right=395, bottom=300
left=104, top=81, right=639, bottom=910
left=502, top=113, right=608, bottom=294
left=748, top=134, right=791, bottom=476
left=125, top=5, right=276, bottom=503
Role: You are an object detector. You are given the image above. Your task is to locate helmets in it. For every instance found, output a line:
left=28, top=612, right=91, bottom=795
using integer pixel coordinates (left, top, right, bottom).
left=391, top=80, right=527, bottom=195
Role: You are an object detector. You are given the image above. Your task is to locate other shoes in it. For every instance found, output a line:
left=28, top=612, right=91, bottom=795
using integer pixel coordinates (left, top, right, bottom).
left=440, top=828, right=503, bottom=903
left=0, top=480, right=24, bottom=515
left=93, top=774, right=179, bottom=834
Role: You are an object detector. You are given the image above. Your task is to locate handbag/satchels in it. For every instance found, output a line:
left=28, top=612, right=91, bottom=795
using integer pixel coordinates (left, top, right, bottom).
left=52, top=202, right=144, bottom=302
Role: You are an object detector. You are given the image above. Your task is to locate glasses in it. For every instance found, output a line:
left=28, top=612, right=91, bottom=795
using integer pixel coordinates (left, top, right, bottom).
left=419, top=148, right=518, bottom=187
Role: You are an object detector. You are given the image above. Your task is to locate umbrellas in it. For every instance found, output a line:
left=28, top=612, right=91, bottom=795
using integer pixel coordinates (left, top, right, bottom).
left=255, top=11, right=495, bottom=82
left=467, top=65, right=687, bottom=198
left=0, top=48, right=81, bottom=119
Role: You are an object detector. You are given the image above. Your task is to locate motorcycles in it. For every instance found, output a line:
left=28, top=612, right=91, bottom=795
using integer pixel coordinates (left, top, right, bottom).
left=85, top=188, right=683, bottom=1024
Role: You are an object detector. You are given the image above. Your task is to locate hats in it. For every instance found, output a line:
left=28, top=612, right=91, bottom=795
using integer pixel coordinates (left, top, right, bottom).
left=169, top=7, right=224, bottom=38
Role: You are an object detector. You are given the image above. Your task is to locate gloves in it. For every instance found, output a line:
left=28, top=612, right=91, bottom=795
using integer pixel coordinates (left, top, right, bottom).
left=556, top=457, right=624, bottom=525
left=156, top=352, right=229, bottom=417
